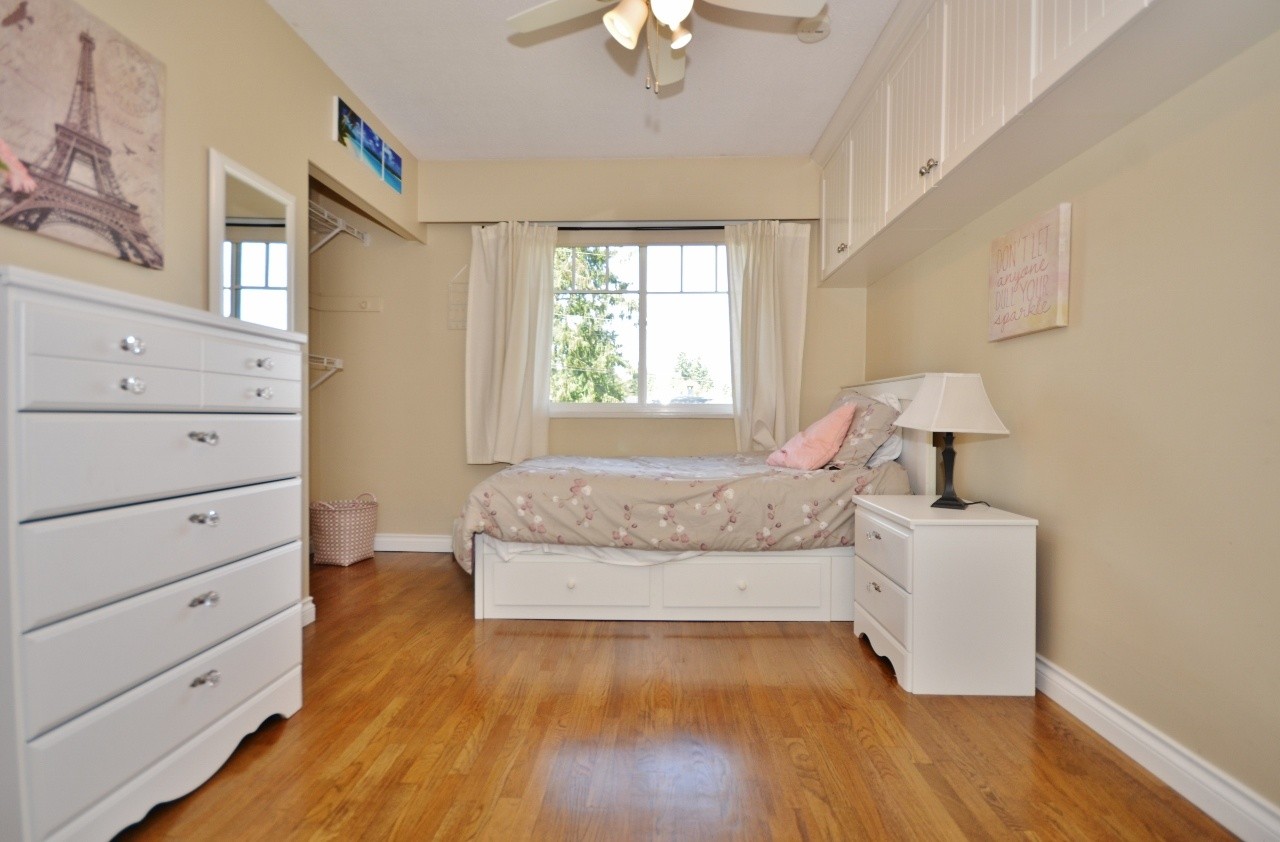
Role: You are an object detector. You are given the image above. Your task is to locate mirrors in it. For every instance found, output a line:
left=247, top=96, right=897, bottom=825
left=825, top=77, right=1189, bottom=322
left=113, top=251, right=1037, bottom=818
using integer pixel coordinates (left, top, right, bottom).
left=205, top=146, right=297, bottom=333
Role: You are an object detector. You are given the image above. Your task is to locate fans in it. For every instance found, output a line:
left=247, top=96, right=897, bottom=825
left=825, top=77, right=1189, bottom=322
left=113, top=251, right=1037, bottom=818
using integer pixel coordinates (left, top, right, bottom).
left=505, top=0, right=828, bottom=86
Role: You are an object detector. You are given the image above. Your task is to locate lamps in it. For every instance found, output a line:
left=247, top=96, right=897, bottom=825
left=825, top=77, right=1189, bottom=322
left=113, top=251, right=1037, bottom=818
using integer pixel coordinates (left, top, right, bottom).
left=601, top=0, right=695, bottom=95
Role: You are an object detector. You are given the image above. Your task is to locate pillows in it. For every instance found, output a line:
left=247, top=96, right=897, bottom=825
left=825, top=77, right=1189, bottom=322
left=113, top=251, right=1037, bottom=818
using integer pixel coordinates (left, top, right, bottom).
left=866, top=392, right=904, bottom=470
left=823, top=389, right=901, bottom=471
left=765, top=401, right=858, bottom=472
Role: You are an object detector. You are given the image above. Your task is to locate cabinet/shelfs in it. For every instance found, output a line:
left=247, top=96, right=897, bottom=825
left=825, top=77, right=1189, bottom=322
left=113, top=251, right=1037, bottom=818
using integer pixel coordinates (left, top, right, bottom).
left=819, top=1, right=1280, bottom=289
left=1, top=260, right=311, bottom=842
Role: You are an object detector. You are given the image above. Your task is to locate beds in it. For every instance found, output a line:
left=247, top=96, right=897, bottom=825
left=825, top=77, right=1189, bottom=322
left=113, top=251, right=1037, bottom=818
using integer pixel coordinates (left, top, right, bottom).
left=452, top=370, right=940, bottom=621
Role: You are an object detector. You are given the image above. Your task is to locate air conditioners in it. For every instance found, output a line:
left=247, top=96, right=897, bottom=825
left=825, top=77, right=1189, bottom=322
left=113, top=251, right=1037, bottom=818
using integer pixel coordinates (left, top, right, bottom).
left=893, top=371, right=1012, bottom=511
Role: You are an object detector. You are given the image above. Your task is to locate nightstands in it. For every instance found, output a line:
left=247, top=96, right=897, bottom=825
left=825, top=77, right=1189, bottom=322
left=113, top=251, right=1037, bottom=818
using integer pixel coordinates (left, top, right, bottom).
left=851, top=491, right=1041, bottom=696
